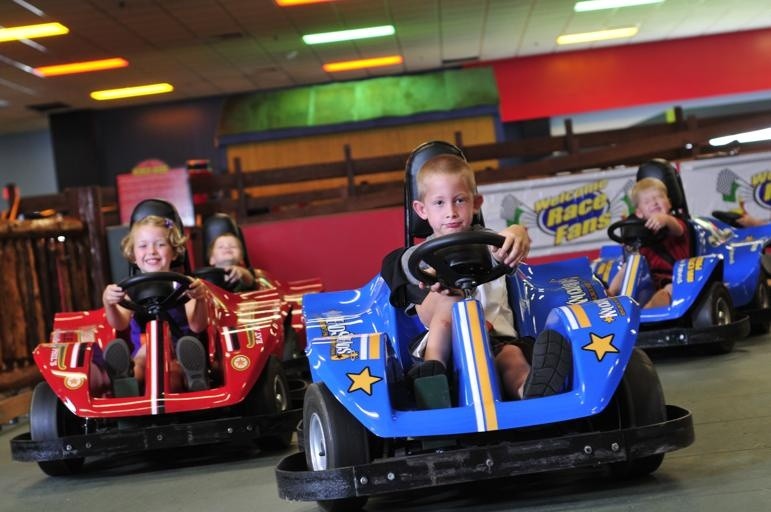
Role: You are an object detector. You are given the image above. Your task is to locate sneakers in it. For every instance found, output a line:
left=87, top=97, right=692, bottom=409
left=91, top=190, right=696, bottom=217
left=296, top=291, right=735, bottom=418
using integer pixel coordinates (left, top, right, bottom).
left=104, top=339, right=134, bottom=396
left=521, top=329, right=573, bottom=400
left=177, top=336, right=208, bottom=391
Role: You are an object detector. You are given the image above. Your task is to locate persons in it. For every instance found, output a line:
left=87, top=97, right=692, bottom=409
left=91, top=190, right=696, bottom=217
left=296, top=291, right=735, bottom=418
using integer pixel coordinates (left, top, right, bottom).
left=382, top=154, right=570, bottom=402
left=206, top=229, right=256, bottom=289
left=594, top=176, right=692, bottom=307
left=103, top=212, right=211, bottom=393
